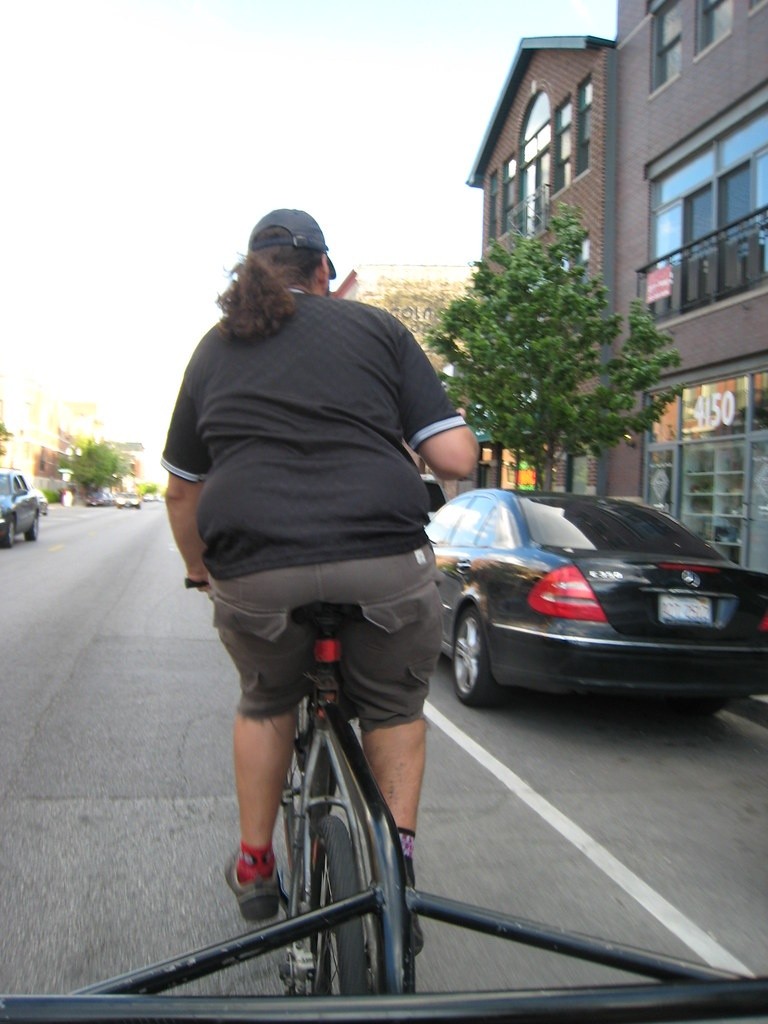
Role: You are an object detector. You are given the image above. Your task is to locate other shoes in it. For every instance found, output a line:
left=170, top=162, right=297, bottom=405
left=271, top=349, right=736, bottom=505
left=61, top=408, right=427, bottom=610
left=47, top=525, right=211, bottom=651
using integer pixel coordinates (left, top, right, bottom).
left=226, top=847, right=278, bottom=921
left=404, top=864, right=424, bottom=954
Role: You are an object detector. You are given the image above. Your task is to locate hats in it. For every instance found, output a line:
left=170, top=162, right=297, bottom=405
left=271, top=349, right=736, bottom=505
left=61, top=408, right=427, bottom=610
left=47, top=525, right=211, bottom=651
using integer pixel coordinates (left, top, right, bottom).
left=248, top=209, right=337, bottom=279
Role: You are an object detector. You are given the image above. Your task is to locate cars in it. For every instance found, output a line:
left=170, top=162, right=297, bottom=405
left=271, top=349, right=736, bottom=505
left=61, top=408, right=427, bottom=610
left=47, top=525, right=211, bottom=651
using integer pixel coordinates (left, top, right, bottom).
left=85, top=488, right=163, bottom=510
left=421, top=475, right=446, bottom=525
left=34, top=488, right=48, bottom=516
left=424, top=488, right=767, bottom=719
left=1, top=468, right=43, bottom=550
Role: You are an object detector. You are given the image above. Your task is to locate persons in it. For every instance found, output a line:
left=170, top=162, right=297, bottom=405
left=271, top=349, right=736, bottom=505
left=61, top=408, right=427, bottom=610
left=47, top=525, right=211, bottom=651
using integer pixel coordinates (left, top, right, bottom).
left=158, top=208, right=477, bottom=957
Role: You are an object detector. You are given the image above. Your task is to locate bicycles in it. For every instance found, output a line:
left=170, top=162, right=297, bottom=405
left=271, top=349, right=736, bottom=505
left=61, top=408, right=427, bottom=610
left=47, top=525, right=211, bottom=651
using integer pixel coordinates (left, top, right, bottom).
left=2, top=600, right=767, bottom=1024
